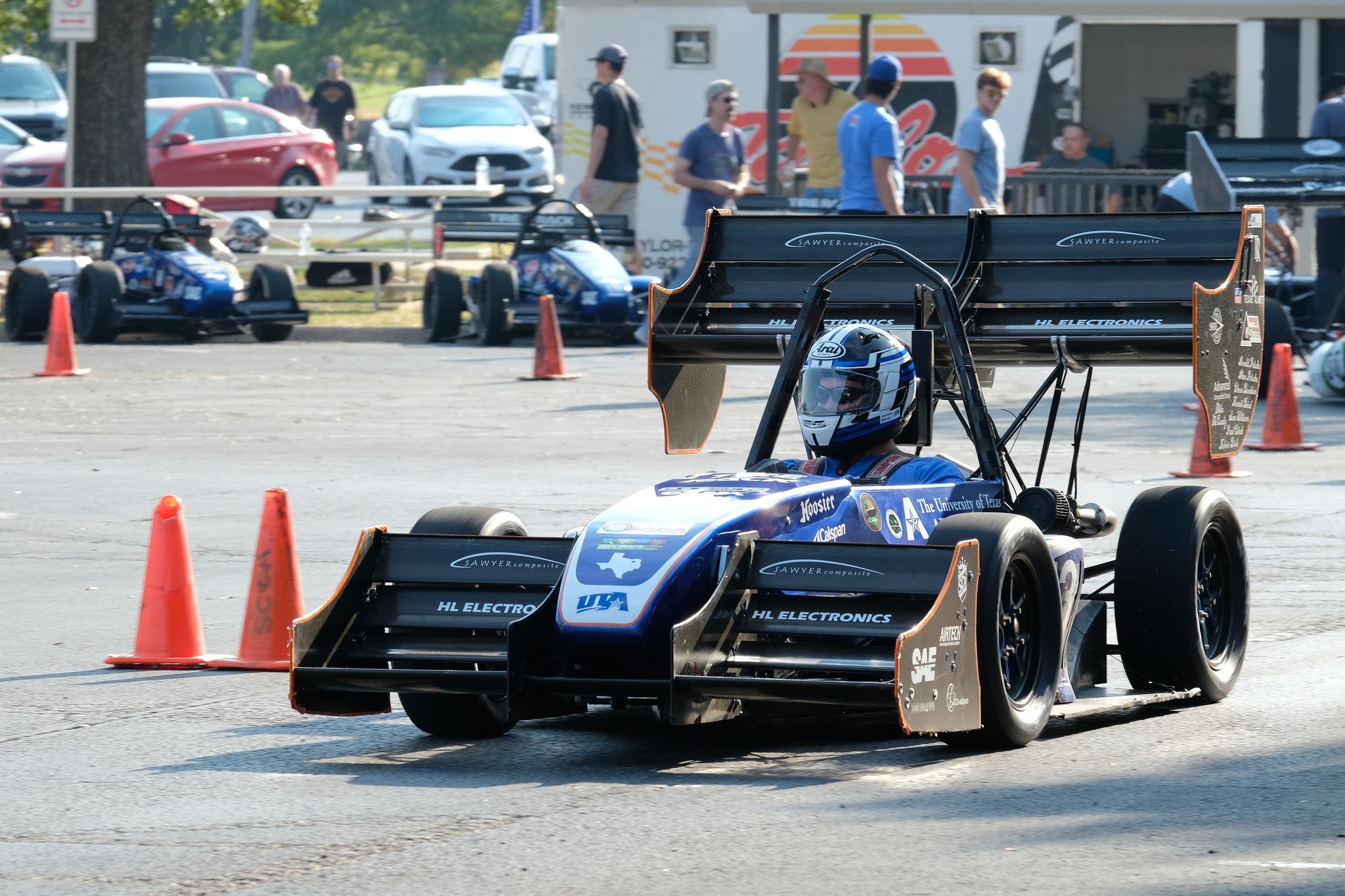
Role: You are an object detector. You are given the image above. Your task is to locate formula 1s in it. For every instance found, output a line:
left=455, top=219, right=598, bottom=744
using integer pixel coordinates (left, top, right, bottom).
left=0, top=194, right=310, bottom=347
left=412, top=194, right=666, bottom=338
left=278, top=200, right=1255, bottom=750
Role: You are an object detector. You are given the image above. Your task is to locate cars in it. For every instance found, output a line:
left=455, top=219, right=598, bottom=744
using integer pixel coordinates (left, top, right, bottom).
left=361, top=80, right=559, bottom=202
left=494, top=31, right=562, bottom=132
left=0, top=52, right=339, bottom=222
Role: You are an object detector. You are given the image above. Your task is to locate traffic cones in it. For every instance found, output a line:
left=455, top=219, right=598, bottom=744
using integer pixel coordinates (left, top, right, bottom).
left=1244, top=342, right=1324, bottom=453
left=202, top=487, right=299, bottom=676
left=520, top=290, right=584, bottom=383
left=107, top=494, right=216, bottom=671
left=1170, top=391, right=1250, bottom=481
left=26, top=289, right=89, bottom=377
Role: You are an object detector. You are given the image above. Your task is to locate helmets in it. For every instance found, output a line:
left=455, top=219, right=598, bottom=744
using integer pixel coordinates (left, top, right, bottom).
left=223, top=214, right=270, bottom=254
left=798, top=325, right=916, bottom=457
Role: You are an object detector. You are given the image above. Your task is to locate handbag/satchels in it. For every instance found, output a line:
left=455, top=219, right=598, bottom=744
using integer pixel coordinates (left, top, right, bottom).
left=306, top=249, right=392, bottom=287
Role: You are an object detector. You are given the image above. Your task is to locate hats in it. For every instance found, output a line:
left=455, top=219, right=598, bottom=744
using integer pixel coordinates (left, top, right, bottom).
left=587, top=44, right=629, bottom=65
left=785, top=57, right=839, bottom=89
left=867, top=55, right=903, bottom=83
left=704, top=78, right=737, bottom=118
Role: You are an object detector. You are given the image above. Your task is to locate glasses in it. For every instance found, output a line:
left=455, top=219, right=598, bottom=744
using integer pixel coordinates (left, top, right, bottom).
left=987, top=89, right=1007, bottom=99
left=813, top=386, right=869, bottom=404
left=721, top=96, right=737, bottom=103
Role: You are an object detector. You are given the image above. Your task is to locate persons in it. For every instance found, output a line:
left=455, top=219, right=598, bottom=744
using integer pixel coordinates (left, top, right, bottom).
left=779, top=322, right=966, bottom=484
left=1156, top=171, right=1296, bottom=274
left=1004, top=122, right=1127, bottom=212
left=299, top=55, right=358, bottom=169
left=577, top=42, right=644, bottom=276
left=262, top=63, right=310, bottom=124
left=786, top=56, right=861, bottom=215
left=835, top=54, right=907, bottom=216
left=635, top=80, right=751, bottom=345
left=1310, top=94, right=1345, bottom=135
left=947, top=68, right=1011, bottom=215
